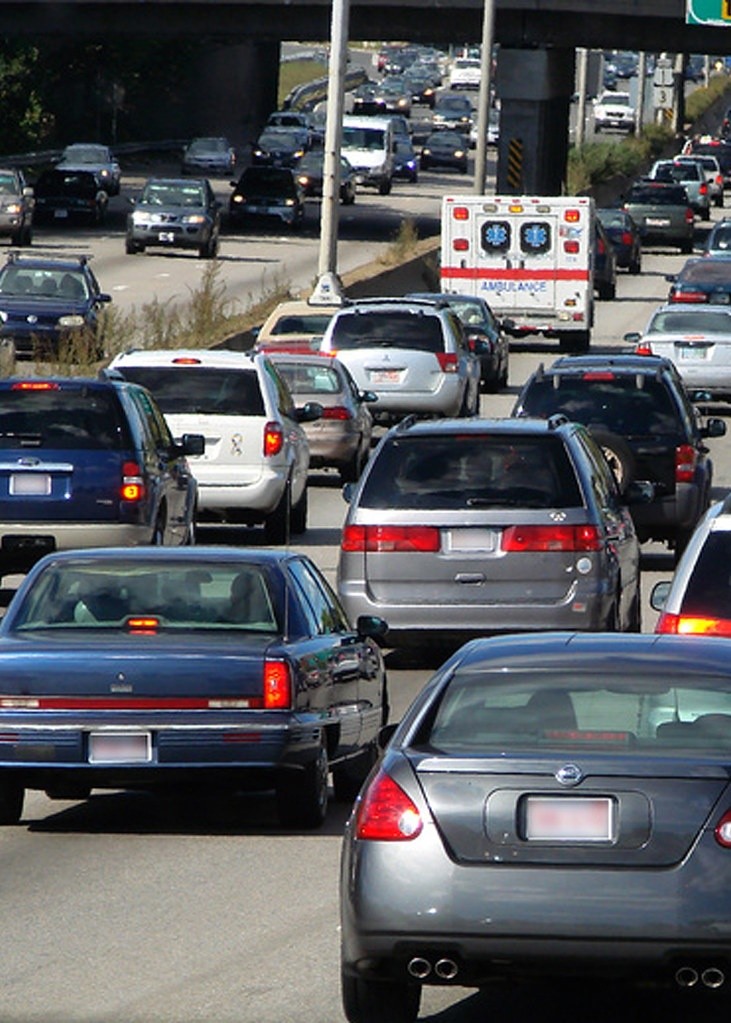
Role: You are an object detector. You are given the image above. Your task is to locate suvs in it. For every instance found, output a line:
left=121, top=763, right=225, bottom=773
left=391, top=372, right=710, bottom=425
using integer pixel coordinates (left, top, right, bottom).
left=336, top=414, right=654, bottom=649
left=637, top=493, right=731, bottom=744
left=105, top=349, right=324, bottom=546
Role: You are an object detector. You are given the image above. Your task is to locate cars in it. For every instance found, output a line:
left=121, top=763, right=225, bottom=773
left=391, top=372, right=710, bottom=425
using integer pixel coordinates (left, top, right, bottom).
left=30, top=170, right=108, bottom=224
left=623, top=216, right=731, bottom=401
left=340, top=46, right=499, bottom=195
left=125, top=178, right=224, bottom=259
left=591, top=51, right=731, bottom=300
left=0, top=250, right=111, bottom=358
left=250, top=292, right=516, bottom=426
left=0, top=376, right=206, bottom=598
left=339, top=632, right=731, bottom=1022
left=50, top=143, right=122, bottom=197
left=269, top=353, right=378, bottom=482
left=510, top=354, right=727, bottom=561
left=228, top=111, right=357, bottom=228
left=0, top=168, right=36, bottom=248
left=0, top=546, right=389, bottom=829
left=181, top=137, right=236, bottom=175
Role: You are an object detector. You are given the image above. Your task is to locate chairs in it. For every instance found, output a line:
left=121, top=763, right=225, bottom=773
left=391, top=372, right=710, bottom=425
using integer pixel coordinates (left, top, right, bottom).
left=455, top=705, right=544, bottom=738
left=11, top=274, right=84, bottom=295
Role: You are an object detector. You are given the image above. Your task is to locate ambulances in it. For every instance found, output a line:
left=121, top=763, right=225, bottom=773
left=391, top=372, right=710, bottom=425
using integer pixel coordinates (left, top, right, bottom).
left=438, top=194, right=596, bottom=352
